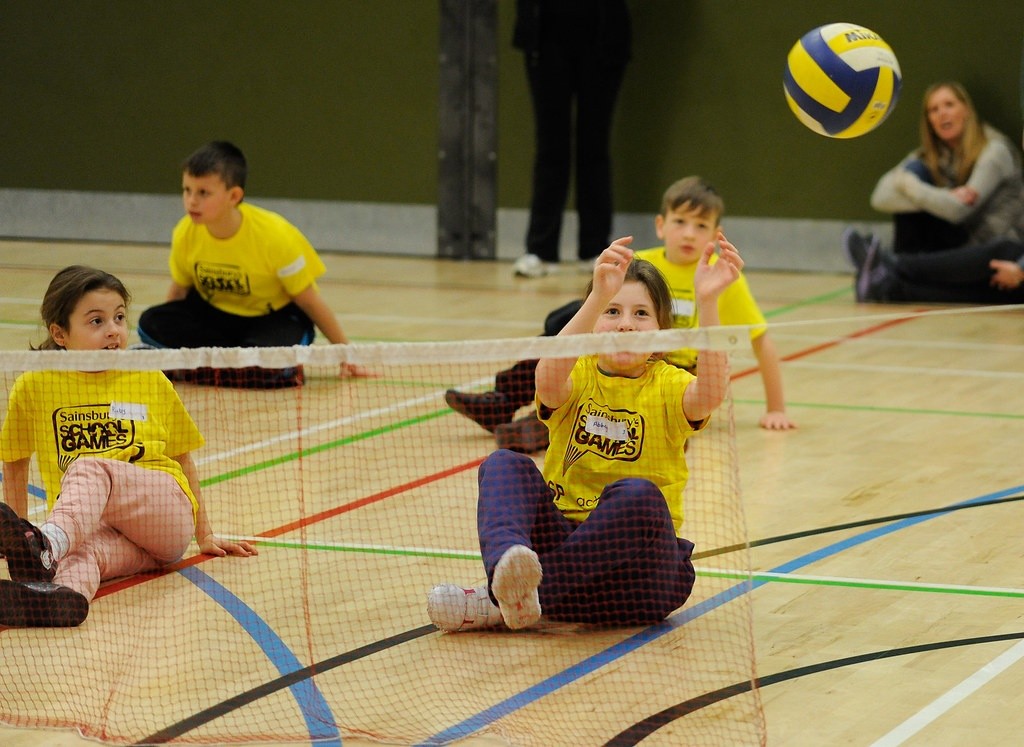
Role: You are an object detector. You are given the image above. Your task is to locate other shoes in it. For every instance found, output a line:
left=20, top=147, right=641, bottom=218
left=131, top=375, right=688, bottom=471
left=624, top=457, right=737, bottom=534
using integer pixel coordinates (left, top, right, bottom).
left=843, top=228, right=864, bottom=284
left=857, top=233, right=897, bottom=303
left=514, top=254, right=558, bottom=277
left=576, top=257, right=599, bottom=271
left=265, top=365, right=303, bottom=388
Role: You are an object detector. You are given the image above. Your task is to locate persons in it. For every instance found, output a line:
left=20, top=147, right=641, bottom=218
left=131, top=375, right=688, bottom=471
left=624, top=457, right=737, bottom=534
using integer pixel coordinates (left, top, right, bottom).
left=444, top=172, right=798, bottom=454
left=511, top=0, right=632, bottom=278
left=424, top=229, right=744, bottom=634
left=847, top=224, right=1024, bottom=305
left=869, top=77, right=1024, bottom=253
left=1, top=264, right=263, bottom=631
left=137, top=138, right=377, bottom=388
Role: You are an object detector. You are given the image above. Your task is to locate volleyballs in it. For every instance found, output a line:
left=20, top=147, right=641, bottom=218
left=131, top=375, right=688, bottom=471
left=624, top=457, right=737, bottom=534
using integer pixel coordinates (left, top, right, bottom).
left=781, top=22, right=903, bottom=140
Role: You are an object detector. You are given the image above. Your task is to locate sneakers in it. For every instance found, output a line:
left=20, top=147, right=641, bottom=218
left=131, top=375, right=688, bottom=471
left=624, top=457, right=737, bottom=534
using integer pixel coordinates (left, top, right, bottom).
left=444, top=390, right=512, bottom=434
left=0, top=503, right=57, bottom=580
left=0, top=580, right=89, bottom=628
left=428, top=583, right=503, bottom=632
left=495, top=412, right=549, bottom=454
left=492, top=545, right=541, bottom=630
